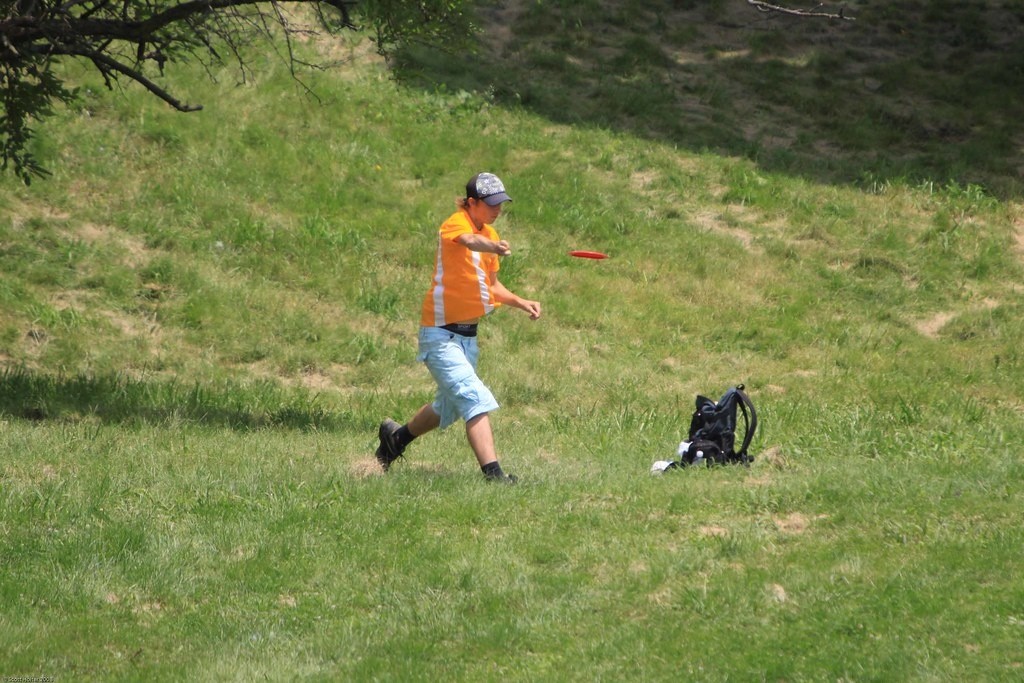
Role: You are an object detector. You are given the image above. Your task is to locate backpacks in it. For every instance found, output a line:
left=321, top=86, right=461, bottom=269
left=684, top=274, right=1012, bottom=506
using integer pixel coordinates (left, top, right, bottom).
left=680, top=384, right=757, bottom=466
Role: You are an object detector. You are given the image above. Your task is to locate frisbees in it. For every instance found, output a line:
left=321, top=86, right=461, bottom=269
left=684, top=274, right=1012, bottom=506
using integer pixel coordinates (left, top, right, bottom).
left=567, top=251, right=609, bottom=259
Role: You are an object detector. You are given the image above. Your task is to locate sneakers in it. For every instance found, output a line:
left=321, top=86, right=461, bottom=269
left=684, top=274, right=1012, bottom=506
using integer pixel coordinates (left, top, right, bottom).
left=375, top=417, right=403, bottom=472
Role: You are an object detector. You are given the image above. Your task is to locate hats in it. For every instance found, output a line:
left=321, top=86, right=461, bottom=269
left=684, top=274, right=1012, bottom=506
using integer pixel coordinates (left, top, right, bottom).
left=465, top=172, right=512, bottom=206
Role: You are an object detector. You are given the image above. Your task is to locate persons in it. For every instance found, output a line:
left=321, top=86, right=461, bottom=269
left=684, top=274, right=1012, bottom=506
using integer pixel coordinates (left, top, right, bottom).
left=375, top=172, right=541, bottom=488
